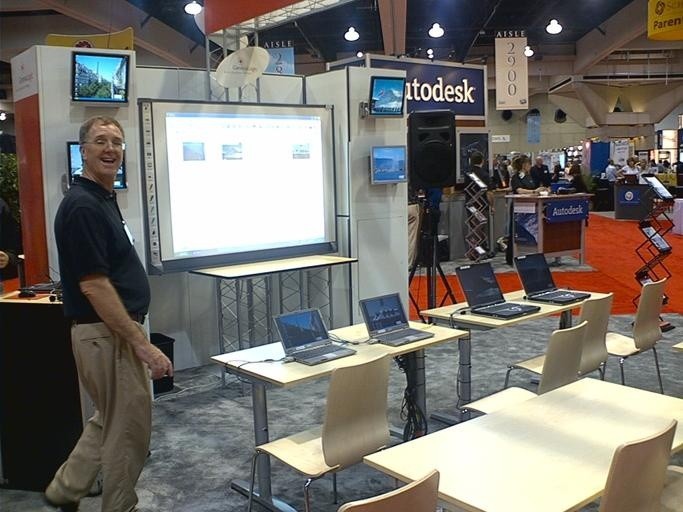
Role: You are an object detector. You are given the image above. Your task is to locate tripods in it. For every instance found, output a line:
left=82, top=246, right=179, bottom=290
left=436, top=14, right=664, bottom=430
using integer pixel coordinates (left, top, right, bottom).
left=409, top=193, right=458, bottom=324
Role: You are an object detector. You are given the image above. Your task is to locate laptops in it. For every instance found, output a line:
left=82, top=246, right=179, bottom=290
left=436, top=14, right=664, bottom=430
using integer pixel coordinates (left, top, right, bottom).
left=513, top=253, right=590, bottom=306
left=271, top=307, right=356, bottom=367
left=359, top=292, right=435, bottom=347
left=16, top=280, right=62, bottom=294
left=454, top=262, right=541, bottom=320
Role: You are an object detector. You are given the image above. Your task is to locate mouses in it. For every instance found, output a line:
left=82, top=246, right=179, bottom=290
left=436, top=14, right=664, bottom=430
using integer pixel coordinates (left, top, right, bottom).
left=19, top=291, right=36, bottom=298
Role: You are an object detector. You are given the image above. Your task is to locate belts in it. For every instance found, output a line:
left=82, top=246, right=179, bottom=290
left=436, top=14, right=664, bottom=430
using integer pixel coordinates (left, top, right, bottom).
left=75, top=311, right=145, bottom=324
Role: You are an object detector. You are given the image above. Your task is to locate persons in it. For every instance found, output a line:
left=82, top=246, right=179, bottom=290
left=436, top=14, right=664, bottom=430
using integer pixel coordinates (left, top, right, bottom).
left=605, top=156, right=683, bottom=184
left=464, top=151, right=496, bottom=259
left=494, top=152, right=589, bottom=196
left=418, top=188, right=451, bottom=266
left=0, top=197, right=22, bottom=283
left=43, top=115, right=173, bottom=511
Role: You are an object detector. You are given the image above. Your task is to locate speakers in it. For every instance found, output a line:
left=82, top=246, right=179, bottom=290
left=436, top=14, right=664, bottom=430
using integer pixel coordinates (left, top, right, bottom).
left=408, top=110, right=457, bottom=188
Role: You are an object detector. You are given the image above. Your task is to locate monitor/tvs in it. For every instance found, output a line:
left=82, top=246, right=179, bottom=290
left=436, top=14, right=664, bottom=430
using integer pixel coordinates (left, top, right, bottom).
left=370, top=145, right=407, bottom=184
left=67, top=140, right=128, bottom=190
left=369, top=75, right=406, bottom=118
left=70, top=50, right=130, bottom=106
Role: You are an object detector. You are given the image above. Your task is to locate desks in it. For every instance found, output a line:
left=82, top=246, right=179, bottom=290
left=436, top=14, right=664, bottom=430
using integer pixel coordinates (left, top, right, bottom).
left=189, top=254, right=359, bottom=354
left=503, top=193, right=594, bottom=265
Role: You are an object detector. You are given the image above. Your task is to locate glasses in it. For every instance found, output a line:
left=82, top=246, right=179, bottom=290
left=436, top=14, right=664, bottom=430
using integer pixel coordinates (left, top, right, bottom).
left=80, top=141, right=122, bottom=147
